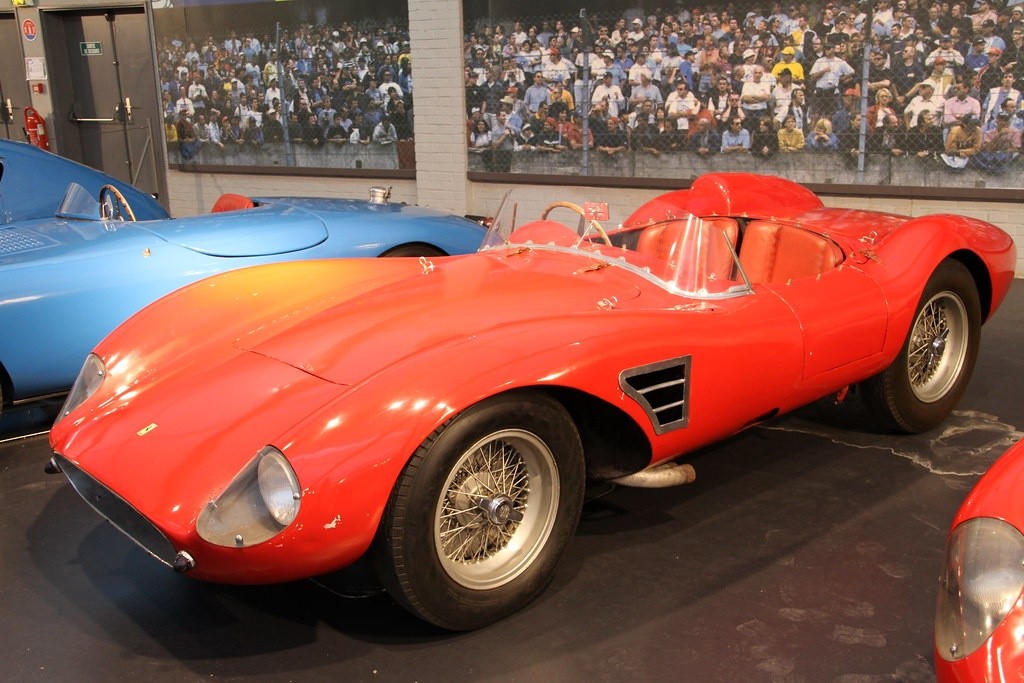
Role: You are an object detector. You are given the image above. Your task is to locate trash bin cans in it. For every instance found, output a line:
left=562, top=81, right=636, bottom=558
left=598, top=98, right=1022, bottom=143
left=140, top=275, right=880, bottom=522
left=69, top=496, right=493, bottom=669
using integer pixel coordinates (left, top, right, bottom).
left=24, top=106, right=49, bottom=152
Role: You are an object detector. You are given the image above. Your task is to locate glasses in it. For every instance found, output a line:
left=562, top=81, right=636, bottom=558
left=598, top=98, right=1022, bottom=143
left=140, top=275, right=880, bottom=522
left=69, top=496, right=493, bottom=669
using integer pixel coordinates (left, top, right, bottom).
left=849, top=18, right=855, bottom=20
left=953, top=86, right=961, bottom=91
left=1012, top=32, right=1021, bottom=35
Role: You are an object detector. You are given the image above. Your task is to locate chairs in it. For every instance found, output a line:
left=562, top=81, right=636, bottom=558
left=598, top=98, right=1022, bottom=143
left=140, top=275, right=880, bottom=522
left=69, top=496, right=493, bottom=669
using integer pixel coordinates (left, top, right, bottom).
left=734, top=220, right=843, bottom=283
left=635, top=216, right=740, bottom=280
left=211, top=193, right=254, bottom=214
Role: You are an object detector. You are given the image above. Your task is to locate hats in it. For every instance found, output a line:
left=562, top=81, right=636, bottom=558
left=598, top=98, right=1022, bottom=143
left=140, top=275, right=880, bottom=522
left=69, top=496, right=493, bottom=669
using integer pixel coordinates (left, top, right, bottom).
left=842, top=88, right=860, bottom=97
left=1012, top=6, right=1023, bottom=14
left=464, top=8, right=729, bottom=132
left=934, top=57, right=944, bottom=64
left=781, top=46, right=795, bottom=55
left=779, top=68, right=791, bottom=76
left=873, top=47, right=887, bottom=58
left=972, top=37, right=985, bottom=46
left=980, top=19, right=994, bottom=27
left=977, top=0, right=992, bottom=4
left=823, top=42, right=836, bottom=48
left=746, top=12, right=756, bottom=19
left=920, top=79, right=937, bottom=89
left=985, top=48, right=1002, bottom=55
left=997, top=111, right=1012, bottom=119
left=904, top=34, right=918, bottom=41
left=962, top=112, right=980, bottom=124
left=940, top=34, right=951, bottom=41
left=179, top=21, right=409, bottom=123
left=742, top=49, right=756, bottom=60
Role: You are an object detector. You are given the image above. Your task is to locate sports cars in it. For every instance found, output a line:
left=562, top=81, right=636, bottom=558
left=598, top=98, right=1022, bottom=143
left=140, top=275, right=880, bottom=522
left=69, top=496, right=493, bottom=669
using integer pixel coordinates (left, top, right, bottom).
left=0, top=183, right=506, bottom=401
left=933, top=438, right=1024, bottom=683
left=42, top=173, right=1017, bottom=632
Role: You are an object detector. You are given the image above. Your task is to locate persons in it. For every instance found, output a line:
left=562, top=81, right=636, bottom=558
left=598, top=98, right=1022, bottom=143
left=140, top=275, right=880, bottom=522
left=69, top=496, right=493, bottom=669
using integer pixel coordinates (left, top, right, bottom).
left=150, top=0, right=1024, bottom=175
